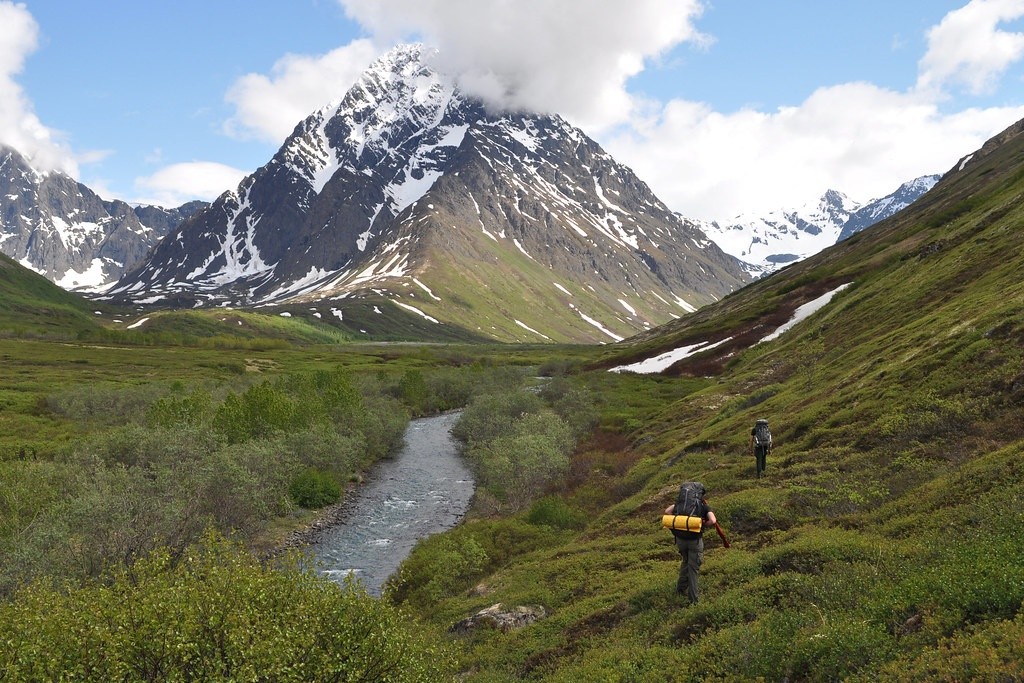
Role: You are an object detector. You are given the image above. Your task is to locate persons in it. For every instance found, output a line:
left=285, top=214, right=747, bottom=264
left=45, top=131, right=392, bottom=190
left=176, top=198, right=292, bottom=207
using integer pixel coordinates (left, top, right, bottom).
left=665, top=483, right=717, bottom=609
left=750, top=420, right=772, bottom=479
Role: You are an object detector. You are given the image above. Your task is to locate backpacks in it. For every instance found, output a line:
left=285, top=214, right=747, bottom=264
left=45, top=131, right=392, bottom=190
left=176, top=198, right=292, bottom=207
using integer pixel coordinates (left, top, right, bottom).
left=755, top=419, right=770, bottom=446
left=673, top=482, right=703, bottom=516
